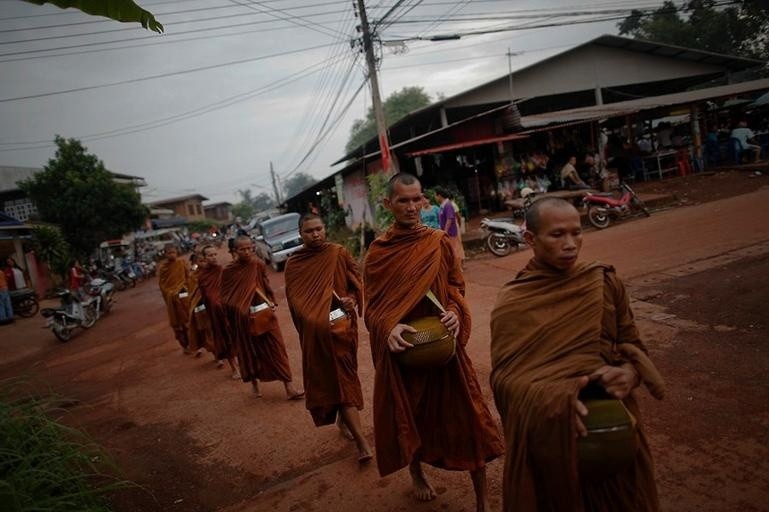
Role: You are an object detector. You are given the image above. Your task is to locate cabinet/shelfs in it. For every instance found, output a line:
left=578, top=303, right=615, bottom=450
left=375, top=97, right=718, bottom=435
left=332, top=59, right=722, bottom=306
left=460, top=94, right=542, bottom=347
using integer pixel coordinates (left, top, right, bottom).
left=642, top=151, right=678, bottom=181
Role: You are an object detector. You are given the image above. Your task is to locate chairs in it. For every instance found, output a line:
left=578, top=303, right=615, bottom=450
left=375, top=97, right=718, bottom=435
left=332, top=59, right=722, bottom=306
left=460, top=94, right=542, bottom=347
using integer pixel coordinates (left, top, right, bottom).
left=728, top=136, right=752, bottom=163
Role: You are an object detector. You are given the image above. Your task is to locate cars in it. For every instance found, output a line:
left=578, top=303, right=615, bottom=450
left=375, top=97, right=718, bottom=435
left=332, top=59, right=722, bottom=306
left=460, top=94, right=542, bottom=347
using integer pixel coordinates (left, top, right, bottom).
left=250, top=212, right=304, bottom=272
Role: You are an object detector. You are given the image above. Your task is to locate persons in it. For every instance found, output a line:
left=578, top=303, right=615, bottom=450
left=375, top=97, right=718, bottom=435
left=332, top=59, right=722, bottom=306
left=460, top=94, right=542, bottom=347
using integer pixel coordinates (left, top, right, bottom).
left=546, top=117, right=763, bottom=194
left=158, top=216, right=305, bottom=403
left=488, top=198, right=667, bottom=512
left=68, top=235, right=156, bottom=301
left=283, top=213, right=372, bottom=462
left=0, top=257, right=30, bottom=326
left=422, top=187, right=463, bottom=271
left=364, top=171, right=504, bottom=512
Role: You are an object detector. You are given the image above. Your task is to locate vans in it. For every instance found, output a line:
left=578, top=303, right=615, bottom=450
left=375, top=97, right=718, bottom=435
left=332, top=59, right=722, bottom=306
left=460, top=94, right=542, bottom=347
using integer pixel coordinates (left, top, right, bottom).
left=99, top=240, right=135, bottom=267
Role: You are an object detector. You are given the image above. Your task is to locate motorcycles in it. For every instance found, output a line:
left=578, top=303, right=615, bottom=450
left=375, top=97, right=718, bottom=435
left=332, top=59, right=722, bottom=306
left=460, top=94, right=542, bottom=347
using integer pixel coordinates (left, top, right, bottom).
left=9, top=257, right=154, bottom=342
left=581, top=175, right=650, bottom=229
left=480, top=192, right=534, bottom=257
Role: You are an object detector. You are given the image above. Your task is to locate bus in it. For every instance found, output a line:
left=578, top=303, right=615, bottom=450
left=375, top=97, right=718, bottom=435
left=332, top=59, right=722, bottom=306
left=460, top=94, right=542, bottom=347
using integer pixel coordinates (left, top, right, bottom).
left=133, top=227, right=192, bottom=257
